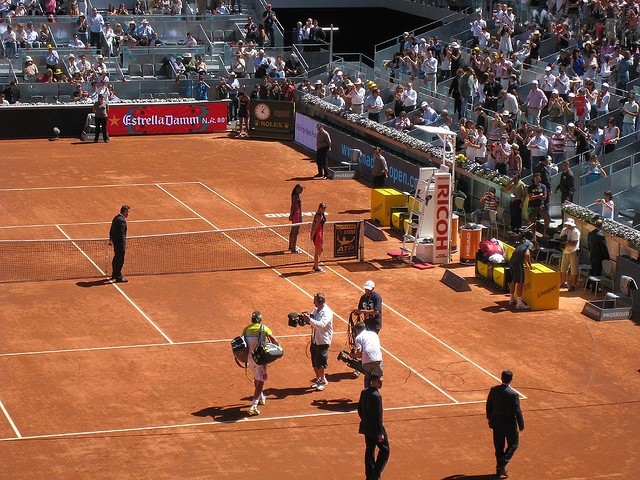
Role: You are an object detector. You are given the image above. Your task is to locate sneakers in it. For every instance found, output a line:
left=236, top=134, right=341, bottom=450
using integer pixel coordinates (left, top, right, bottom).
left=315, top=267, right=323, bottom=272
left=352, top=370, right=360, bottom=377
left=517, top=302, right=531, bottom=309
left=568, top=285, right=575, bottom=291
left=317, top=380, right=328, bottom=390
left=104, top=139, right=108, bottom=143
left=509, top=299, right=517, bottom=305
left=560, top=283, right=569, bottom=287
left=311, top=379, right=320, bottom=387
left=260, top=396, right=267, bottom=405
left=248, top=407, right=260, bottom=415
left=314, top=174, right=323, bottom=178
left=93, top=139, right=99, bottom=143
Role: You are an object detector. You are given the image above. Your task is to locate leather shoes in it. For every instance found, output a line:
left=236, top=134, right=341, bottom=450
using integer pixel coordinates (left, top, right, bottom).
left=116, top=277, right=128, bottom=282
left=497, top=468, right=507, bottom=476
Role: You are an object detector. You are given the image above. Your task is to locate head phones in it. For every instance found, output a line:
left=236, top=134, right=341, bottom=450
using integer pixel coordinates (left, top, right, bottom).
left=316, top=292, right=323, bottom=304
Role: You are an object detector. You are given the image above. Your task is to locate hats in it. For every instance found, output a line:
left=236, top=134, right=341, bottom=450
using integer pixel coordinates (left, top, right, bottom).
left=236, top=40, right=245, bottom=46
left=364, top=280, right=375, bottom=290
left=67, top=54, right=76, bottom=59
left=199, top=75, right=205, bottom=79
left=296, top=184, right=306, bottom=189
left=354, top=78, right=363, bottom=85
left=47, top=44, right=53, bottom=49
left=450, top=42, right=460, bottom=49
left=556, top=126, right=563, bottom=133
left=228, top=72, right=238, bottom=78
left=73, top=69, right=80, bottom=74
left=531, top=30, right=542, bottom=37
left=314, top=80, right=323, bottom=85
left=511, top=143, right=519, bottom=150
left=248, top=41, right=254, bottom=46
left=532, top=79, right=539, bottom=85
left=98, top=94, right=104, bottom=97
left=24, top=56, right=34, bottom=63
left=568, top=123, right=575, bottom=127
left=183, top=53, right=192, bottom=57
left=512, top=172, right=520, bottom=178
left=501, top=134, right=509, bottom=139
left=79, top=12, right=84, bottom=16
left=477, top=125, right=484, bottom=131
left=545, top=66, right=551, bottom=71
left=141, top=18, right=149, bottom=23
left=563, top=218, right=577, bottom=227
left=583, top=41, right=595, bottom=49
left=552, top=89, right=558, bottom=95
left=98, top=54, right=105, bottom=59
left=176, top=56, right=184, bottom=60
left=315, top=123, right=324, bottom=128
left=332, top=66, right=341, bottom=74
left=76, top=83, right=81, bottom=87
left=267, top=4, right=272, bottom=7
left=128, top=20, right=137, bottom=27
left=236, top=90, right=243, bottom=97
left=54, top=68, right=63, bottom=74
left=472, top=47, right=481, bottom=52
left=421, top=101, right=428, bottom=107
left=320, top=203, right=327, bottom=208
left=502, top=110, right=510, bottom=116
left=604, top=54, right=613, bottom=59
left=570, top=77, right=582, bottom=82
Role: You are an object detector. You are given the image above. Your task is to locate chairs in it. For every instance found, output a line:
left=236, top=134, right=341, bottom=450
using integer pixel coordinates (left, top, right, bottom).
left=584, top=259, right=617, bottom=299
left=535, top=247, right=591, bottom=285
left=339, top=149, right=362, bottom=178
left=472, top=206, right=505, bottom=240
left=128, top=60, right=168, bottom=80
left=205, top=29, right=237, bottom=48
left=138, top=91, right=179, bottom=98
left=604, top=275, right=638, bottom=308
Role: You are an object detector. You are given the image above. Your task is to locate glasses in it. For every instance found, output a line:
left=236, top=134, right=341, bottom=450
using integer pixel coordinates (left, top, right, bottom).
left=73, top=36, right=78, bottom=38
left=373, top=380, right=384, bottom=385
left=609, top=121, right=612, bottom=123
left=397, top=88, right=402, bottom=89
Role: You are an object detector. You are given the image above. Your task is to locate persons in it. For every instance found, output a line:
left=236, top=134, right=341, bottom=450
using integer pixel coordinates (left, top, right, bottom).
left=14, top=3, right=28, bottom=17
left=196, top=55, right=208, bottom=75
left=617, top=91, right=636, bottom=108
left=603, top=116, right=620, bottom=153
left=545, top=156, right=558, bottom=174
left=595, top=82, right=610, bottom=118
left=508, top=143, right=523, bottom=181
left=303, top=19, right=314, bottom=43
left=384, top=108, right=394, bottom=121
left=458, top=67, right=480, bottom=116
left=469, top=2, right=540, bottom=72
left=171, top=0, right=182, bottom=14
left=313, top=81, right=324, bottom=98
left=106, top=3, right=119, bottom=16
left=77, top=55, right=91, bottom=69
left=568, top=88, right=591, bottom=121
left=540, top=0, right=640, bottom=74
left=72, top=82, right=87, bottom=99
left=355, top=279, right=383, bottom=336
left=356, top=375, right=391, bottom=479
left=228, top=71, right=240, bottom=121
left=483, top=369, right=526, bottom=478
left=344, top=77, right=351, bottom=86
left=527, top=172, right=550, bottom=239
left=231, top=0, right=243, bottom=15
left=574, top=120, right=588, bottom=140
left=126, top=20, right=137, bottom=33
left=245, top=41, right=256, bottom=78
left=22, top=55, right=38, bottom=81
left=373, top=146, right=390, bottom=189
left=215, top=77, right=231, bottom=99
left=57, top=1, right=66, bottom=15
left=524, top=80, right=548, bottom=126
left=260, top=78, right=272, bottom=100
left=138, top=34, right=151, bottom=46
left=93, top=94, right=111, bottom=143
left=266, top=57, right=275, bottom=76
left=263, top=3, right=277, bottom=47
left=114, top=24, right=124, bottom=36
left=393, top=82, right=404, bottom=115
left=259, top=22, right=269, bottom=46
left=107, top=83, right=118, bottom=102
left=534, top=155, right=556, bottom=223
left=119, top=4, right=131, bottom=14
left=149, top=0, right=162, bottom=14
left=555, top=160, right=576, bottom=227
left=547, top=88, right=568, bottom=122
left=400, top=81, right=418, bottom=111
left=150, top=32, right=163, bottom=46
left=502, top=172, right=527, bottom=233
left=465, top=119, right=475, bottom=159
left=622, top=97, right=639, bottom=134
left=493, top=110, right=514, bottom=130
left=300, top=78, right=314, bottom=94
left=82, top=89, right=90, bottom=102
left=3, top=80, right=20, bottom=101
left=286, top=52, right=300, bottom=76
left=363, top=87, right=384, bottom=122
left=251, top=83, right=259, bottom=100
left=566, top=119, right=590, bottom=162
left=26, top=0, right=42, bottom=15
left=236, top=91, right=249, bottom=138
left=43, top=67, right=57, bottom=83
left=132, top=0, right=145, bottom=14
left=344, top=76, right=365, bottom=115
left=193, top=75, right=212, bottom=100
left=68, top=34, right=86, bottom=48
left=515, top=119, right=531, bottom=168
left=527, top=127, right=549, bottom=171
left=77, top=12, right=87, bottom=31
left=364, top=78, right=378, bottom=95
left=482, top=72, right=503, bottom=111
left=0, top=0, right=13, bottom=17
left=560, top=217, right=581, bottom=292
left=419, top=101, right=438, bottom=124
left=554, top=67, right=570, bottom=95
left=282, top=79, right=294, bottom=100
left=102, top=22, right=116, bottom=56
left=70, top=69, right=82, bottom=82
left=314, top=122, right=335, bottom=182
left=16, top=23, right=27, bottom=48
left=568, top=73, right=582, bottom=94
left=162, top=0, right=171, bottom=14
left=475, top=186, right=500, bottom=226
left=69, top=0, right=83, bottom=16
left=44, top=44, right=60, bottom=70
left=99, top=74, right=110, bottom=95
left=595, top=191, right=615, bottom=220
left=302, top=292, right=334, bottom=393
left=539, top=67, right=555, bottom=103
left=0, top=93, right=12, bottom=106
left=587, top=218, right=610, bottom=276
left=327, top=67, right=340, bottom=84
left=90, top=80, right=100, bottom=97
left=493, top=134, right=511, bottom=175
left=95, top=54, right=107, bottom=74
left=331, top=88, right=344, bottom=106
left=506, top=72, right=519, bottom=91
left=182, top=32, right=198, bottom=47
left=448, top=68, right=463, bottom=117
left=66, top=53, right=77, bottom=71
left=584, top=78, right=597, bottom=109
left=53, top=68, right=68, bottom=83
left=44, top=0, right=57, bottom=15
left=120, top=33, right=137, bottom=47
left=250, top=23, right=258, bottom=40
left=310, top=202, right=328, bottom=274
left=508, top=239, right=539, bottom=310
left=240, top=309, right=282, bottom=416
left=87, top=7, right=105, bottom=54
left=137, top=18, right=155, bottom=36
left=499, top=119, right=517, bottom=149
left=243, top=16, right=257, bottom=39
left=47, top=14, right=56, bottom=24
left=395, top=110, right=411, bottom=131
left=270, top=80, right=282, bottom=99
left=38, top=24, right=53, bottom=48
left=83, top=67, right=97, bottom=81
left=252, top=49, right=267, bottom=77
left=293, top=21, right=303, bottom=41
left=351, top=320, right=384, bottom=387
left=173, top=55, right=186, bottom=77
left=382, top=30, right=462, bottom=79
left=337, top=71, right=342, bottom=86
left=182, top=52, right=199, bottom=78
left=217, top=1, right=229, bottom=15
left=26, top=24, right=38, bottom=47
left=551, top=124, right=566, bottom=158
left=288, top=183, right=305, bottom=253
left=2, top=24, right=16, bottom=59
left=586, top=122, right=602, bottom=153
left=310, top=20, right=326, bottom=51
left=275, top=54, right=286, bottom=77
left=463, top=126, right=487, bottom=163
left=497, top=89, right=519, bottom=114
left=439, top=117, right=454, bottom=144
left=232, top=39, right=251, bottom=78
left=583, top=155, right=607, bottom=185
left=208, top=6, right=223, bottom=15
left=109, top=205, right=131, bottom=283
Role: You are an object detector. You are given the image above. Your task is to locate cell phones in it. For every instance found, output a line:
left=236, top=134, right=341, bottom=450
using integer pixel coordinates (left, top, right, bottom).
left=497, top=138, right=501, bottom=145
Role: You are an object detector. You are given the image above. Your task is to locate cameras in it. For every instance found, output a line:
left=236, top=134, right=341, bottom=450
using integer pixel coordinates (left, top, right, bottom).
left=561, top=234, right=568, bottom=241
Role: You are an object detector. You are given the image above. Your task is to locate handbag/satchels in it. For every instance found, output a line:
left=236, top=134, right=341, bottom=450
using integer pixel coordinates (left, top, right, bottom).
left=252, top=341, right=283, bottom=365
left=480, top=239, right=503, bottom=258
left=231, top=335, right=249, bottom=363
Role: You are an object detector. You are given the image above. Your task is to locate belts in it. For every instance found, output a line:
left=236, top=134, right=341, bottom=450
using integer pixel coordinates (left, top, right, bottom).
left=426, top=72, right=437, bottom=75
left=353, top=103, right=364, bottom=106
